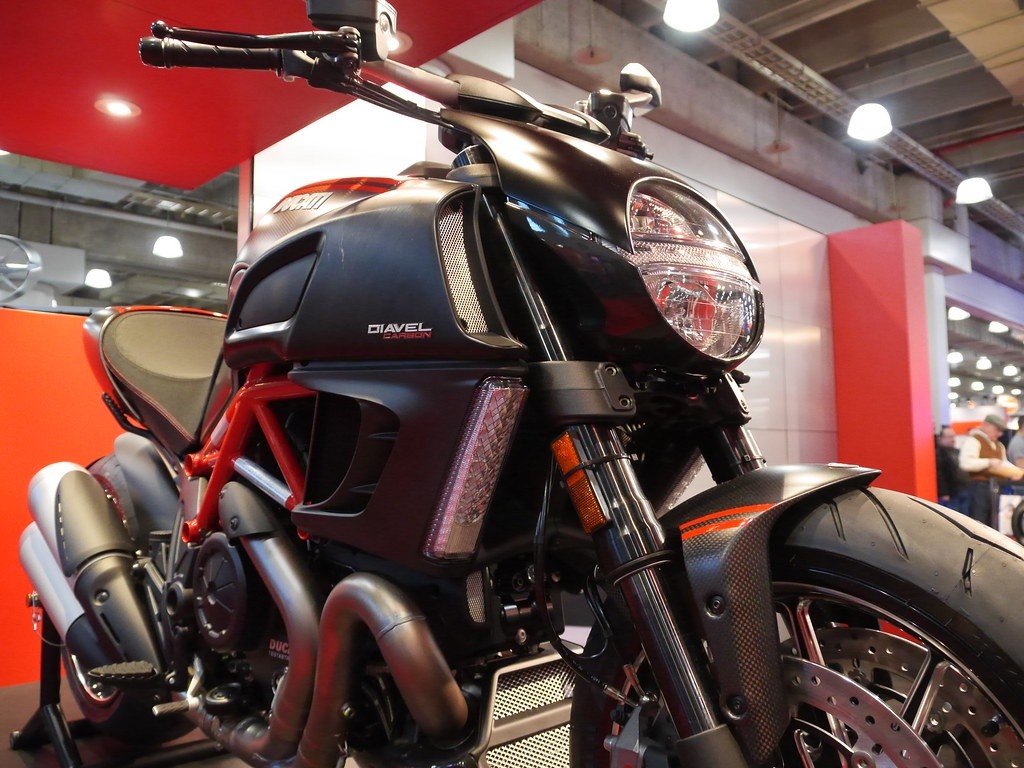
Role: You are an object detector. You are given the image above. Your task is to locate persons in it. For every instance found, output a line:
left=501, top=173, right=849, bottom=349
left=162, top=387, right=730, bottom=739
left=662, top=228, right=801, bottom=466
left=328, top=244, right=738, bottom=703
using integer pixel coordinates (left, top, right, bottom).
left=1007, top=415, right=1024, bottom=469
left=935, top=425, right=961, bottom=506
left=959, top=415, right=1008, bottom=530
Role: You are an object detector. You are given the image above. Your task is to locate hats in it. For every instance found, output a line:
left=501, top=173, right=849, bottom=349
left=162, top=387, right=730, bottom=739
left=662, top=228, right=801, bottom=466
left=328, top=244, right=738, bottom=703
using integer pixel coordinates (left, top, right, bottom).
left=984, top=414, right=1009, bottom=431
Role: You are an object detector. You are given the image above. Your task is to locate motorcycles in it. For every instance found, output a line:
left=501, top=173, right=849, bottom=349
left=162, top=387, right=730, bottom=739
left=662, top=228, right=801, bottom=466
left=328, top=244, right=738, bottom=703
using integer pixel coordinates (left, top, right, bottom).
left=6, top=0, right=1022, bottom=768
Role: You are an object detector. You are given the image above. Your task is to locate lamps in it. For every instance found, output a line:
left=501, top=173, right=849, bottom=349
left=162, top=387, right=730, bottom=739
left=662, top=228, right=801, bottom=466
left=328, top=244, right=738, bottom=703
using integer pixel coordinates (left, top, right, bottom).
left=152, top=206, right=183, bottom=258
left=84, top=268, right=113, bottom=288
left=955, top=134, right=993, bottom=204
left=573, top=1, right=610, bottom=65
left=846, top=14, right=893, bottom=140
left=662, top=0, right=719, bottom=33
left=765, top=97, right=791, bottom=155
